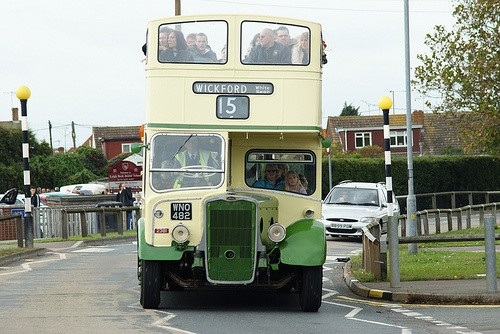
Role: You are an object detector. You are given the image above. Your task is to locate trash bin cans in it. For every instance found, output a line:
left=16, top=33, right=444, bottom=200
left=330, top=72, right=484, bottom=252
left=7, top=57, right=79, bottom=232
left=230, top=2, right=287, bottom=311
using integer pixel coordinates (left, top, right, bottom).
left=96, top=202, right=122, bottom=233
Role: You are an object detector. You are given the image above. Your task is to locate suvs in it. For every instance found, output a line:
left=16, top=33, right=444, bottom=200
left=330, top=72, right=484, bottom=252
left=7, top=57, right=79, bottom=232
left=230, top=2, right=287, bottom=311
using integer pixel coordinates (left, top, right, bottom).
left=322, top=179, right=401, bottom=242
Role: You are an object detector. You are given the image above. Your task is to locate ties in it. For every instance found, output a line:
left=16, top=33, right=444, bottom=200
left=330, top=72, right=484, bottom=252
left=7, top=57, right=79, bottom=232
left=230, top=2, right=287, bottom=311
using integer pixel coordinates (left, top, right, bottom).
left=191, top=154, right=195, bottom=160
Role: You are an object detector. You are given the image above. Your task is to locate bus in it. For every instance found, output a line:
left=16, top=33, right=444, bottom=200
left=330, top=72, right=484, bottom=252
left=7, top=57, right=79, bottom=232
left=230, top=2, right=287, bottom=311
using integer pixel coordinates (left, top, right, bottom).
left=134, top=12, right=328, bottom=313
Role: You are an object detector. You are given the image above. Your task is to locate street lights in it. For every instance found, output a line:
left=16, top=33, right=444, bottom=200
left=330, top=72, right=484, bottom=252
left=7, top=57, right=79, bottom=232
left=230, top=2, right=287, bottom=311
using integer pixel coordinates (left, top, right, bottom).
left=378, top=96, right=394, bottom=218
left=16, top=85, right=36, bottom=250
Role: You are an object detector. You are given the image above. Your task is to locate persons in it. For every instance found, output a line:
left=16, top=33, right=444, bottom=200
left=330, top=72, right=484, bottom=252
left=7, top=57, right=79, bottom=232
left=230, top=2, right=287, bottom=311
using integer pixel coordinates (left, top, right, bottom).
left=36, top=186, right=136, bottom=202
left=243, top=25, right=329, bottom=66
left=252, top=164, right=284, bottom=192
left=30, top=186, right=44, bottom=238
left=285, top=171, right=307, bottom=195
left=278, top=164, right=290, bottom=175
left=115, top=184, right=133, bottom=230
left=173, top=136, right=219, bottom=189
left=151, top=160, right=174, bottom=187
left=141, top=26, right=230, bottom=65
left=135, top=191, right=141, bottom=202
left=298, top=174, right=314, bottom=196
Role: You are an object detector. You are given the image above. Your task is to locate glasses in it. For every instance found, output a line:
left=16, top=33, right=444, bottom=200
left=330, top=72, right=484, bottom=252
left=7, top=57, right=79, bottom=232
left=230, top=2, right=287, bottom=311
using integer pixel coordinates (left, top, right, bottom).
left=266, top=170, right=276, bottom=172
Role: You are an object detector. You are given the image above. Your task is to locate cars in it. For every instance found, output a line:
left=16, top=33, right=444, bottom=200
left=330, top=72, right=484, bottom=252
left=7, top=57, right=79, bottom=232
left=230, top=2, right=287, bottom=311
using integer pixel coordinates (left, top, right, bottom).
left=0, top=183, right=108, bottom=238
